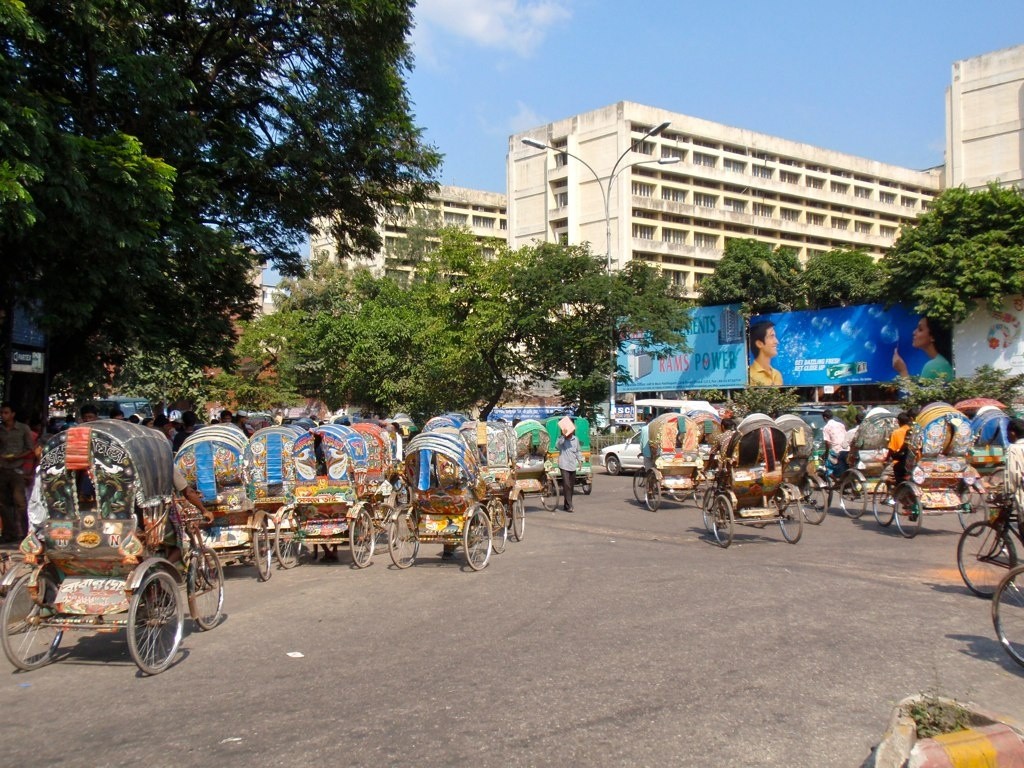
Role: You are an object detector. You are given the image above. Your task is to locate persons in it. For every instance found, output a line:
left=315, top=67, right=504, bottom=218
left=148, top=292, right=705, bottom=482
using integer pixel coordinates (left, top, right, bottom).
left=0, top=402, right=521, bottom=603
left=892, top=316, right=953, bottom=387
left=644, top=414, right=654, bottom=426
left=1003, top=419, right=1024, bottom=545
left=821, top=402, right=920, bottom=505
left=555, top=428, right=582, bottom=512
left=705, top=419, right=737, bottom=473
left=747, top=321, right=783, bottom=386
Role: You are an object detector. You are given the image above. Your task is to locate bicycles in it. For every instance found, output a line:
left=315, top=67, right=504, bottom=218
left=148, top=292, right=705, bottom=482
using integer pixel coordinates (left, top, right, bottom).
left=956, top=467, right=1024, bottom=599
left=991, top=565, right=1024, bottom=668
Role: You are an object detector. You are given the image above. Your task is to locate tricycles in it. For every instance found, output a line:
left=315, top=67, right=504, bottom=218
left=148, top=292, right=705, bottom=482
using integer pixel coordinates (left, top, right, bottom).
left=247, top=422, right=310, bottom=560
left=275, top=423, right=395, bottom=570
left=773, top=398, right=1024, bottom=540
left=206, top=405, right=594, bottom=542
left=174, top=422, right=272, bottom=582
left=388, top=425, right=508, bottom=571
left=631, top=412, right=712, bottom=512
left=0, top=418, right=224, bottom=676
left=701, top=413, right=804, bottom=548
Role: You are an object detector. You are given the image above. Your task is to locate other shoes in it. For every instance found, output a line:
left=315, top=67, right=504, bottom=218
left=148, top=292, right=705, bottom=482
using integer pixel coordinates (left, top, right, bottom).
left=568, top=508, right=574, bottom=512
left=442, top=552, right=456, bottom=560
left=881, top=498, right=893, bottom=505
left=320, top=555, right=339, bottom=563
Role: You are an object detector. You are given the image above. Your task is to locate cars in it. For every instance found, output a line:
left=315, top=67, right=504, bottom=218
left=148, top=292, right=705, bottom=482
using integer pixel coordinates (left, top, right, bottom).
left=598, top=430, right=646, bottom=475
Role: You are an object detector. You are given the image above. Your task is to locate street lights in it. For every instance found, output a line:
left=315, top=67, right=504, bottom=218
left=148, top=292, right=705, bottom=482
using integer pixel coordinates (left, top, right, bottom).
left=520, top=120, right=681, bottom=431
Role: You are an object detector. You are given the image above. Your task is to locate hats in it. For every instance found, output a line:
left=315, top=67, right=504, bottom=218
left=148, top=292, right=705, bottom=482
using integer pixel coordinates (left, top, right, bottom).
left=237, top=410, right=247, bottom=416
